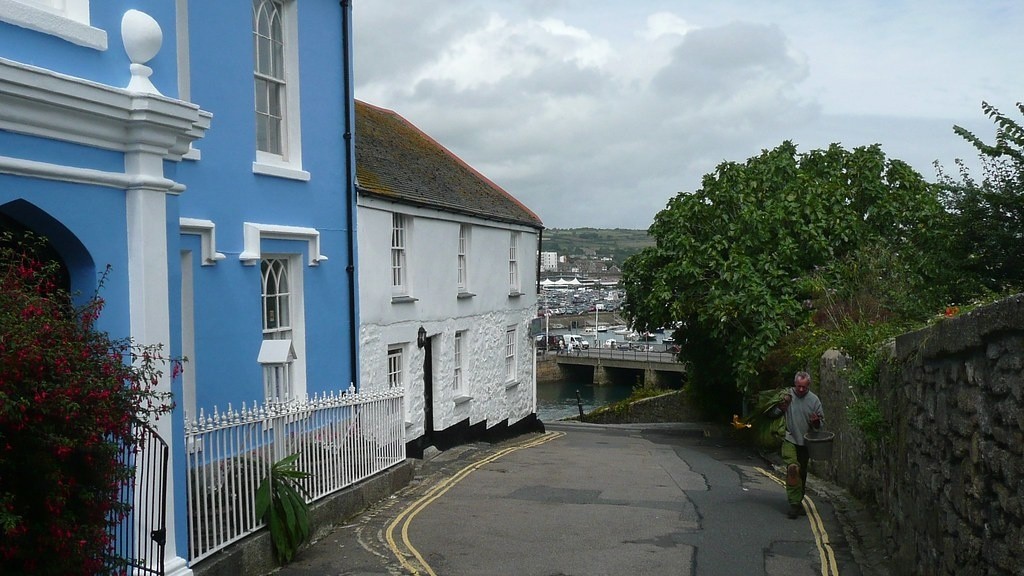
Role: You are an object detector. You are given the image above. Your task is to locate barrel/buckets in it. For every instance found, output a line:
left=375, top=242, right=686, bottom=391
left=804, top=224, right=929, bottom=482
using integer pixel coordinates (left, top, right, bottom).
left=803, top=418, right=835, bottom=460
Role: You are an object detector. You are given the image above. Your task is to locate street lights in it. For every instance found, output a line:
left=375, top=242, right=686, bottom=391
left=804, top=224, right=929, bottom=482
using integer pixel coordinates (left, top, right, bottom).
left=594, top=303, right=604, bottom=348
left=545, top=309, right=553, bottom=352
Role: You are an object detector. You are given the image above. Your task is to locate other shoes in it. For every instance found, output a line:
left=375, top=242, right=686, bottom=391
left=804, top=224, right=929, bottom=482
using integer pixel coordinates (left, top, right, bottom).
left=788, top=505, right=800, bottom=518
left=800, top=505, right=806, bottom=515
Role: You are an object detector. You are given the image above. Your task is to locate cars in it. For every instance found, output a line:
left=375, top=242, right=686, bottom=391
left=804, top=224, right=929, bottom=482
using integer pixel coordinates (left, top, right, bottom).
left=537, top=284, right=684, bottom=352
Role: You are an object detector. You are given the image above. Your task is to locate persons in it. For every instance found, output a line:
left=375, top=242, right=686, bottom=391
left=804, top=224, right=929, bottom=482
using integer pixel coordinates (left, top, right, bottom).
left=559, top=339, right=565, bottom=354
left=773, top=371, right=824, bottom=518
left=568, top=341, right=573, bottom=354
